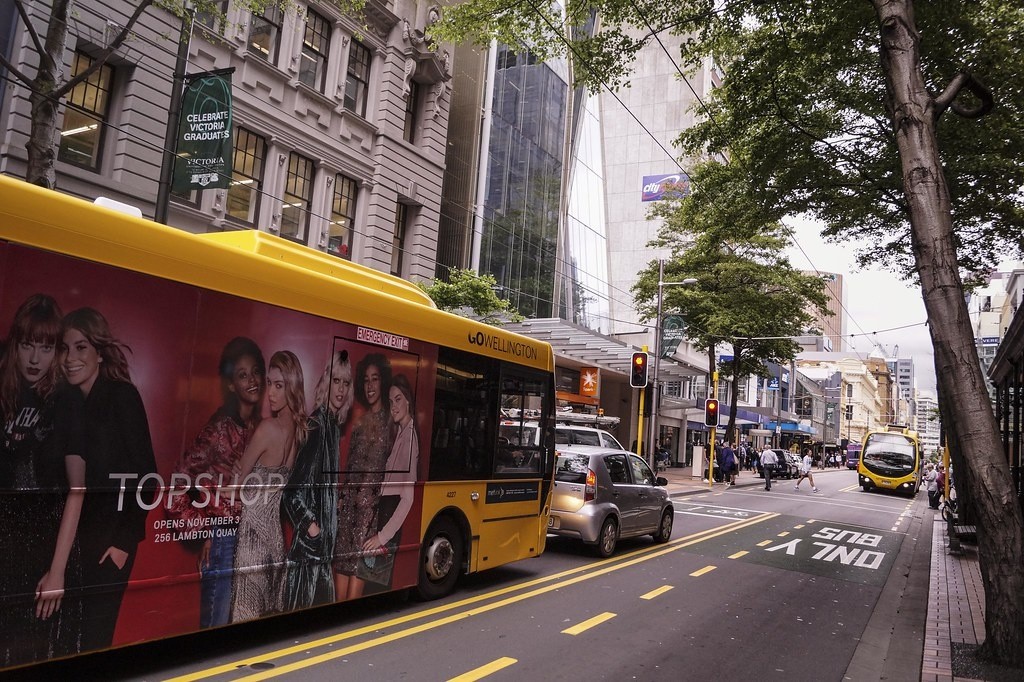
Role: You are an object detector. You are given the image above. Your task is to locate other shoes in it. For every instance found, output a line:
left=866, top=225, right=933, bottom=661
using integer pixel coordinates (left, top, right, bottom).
left=813, top=488, right=820, bottom=493
left=730, top=481, right=735, bottom=485
left=765, top=488, right=770, bottom=491
left=929, top=507, right=933, bottom=509
left=794, top=487, right=799, bottom=490
left=934, top=508, right=938, bottom=509
left=703, top=479, right=709, bottom=483
left=938, top=502, right=941, bottom=506
left=712, top=478, right=716, bottom=482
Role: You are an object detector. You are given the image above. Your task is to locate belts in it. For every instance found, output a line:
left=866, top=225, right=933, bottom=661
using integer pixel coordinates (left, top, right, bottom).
left=764, top=464, right=773, bottom=465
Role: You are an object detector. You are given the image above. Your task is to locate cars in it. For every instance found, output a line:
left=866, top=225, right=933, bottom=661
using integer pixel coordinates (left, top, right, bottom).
left=791, top=454, right=803, bottom=473
left=533, top=443, right=676, bottom=557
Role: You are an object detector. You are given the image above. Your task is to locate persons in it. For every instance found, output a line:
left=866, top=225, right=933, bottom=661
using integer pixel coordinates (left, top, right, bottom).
left=687, top=437, right=762, bottom=485
left=922, top=464, right=946, bottom=510
left=760, top=445, right=778, bottom=490
left=815, top=452, right=846, bottom=470
left=0, top=292, right=154, bottom=667
left=497, top=447, right=524, bottom=462
left=632, top=434, right=660, bottom=472
left=794, top=448, right=821, bottom=493
left=162, top=335, right=419, bottom=631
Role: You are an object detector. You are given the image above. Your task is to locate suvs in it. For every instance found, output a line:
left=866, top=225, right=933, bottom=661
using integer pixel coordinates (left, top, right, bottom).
left=757, top=450, right=799, bottom=479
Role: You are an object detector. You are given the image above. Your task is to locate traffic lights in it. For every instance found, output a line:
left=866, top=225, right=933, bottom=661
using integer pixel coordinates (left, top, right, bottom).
left=703, top=398, right=720, bottom=427
left=629, top=352, right=648, bottom=388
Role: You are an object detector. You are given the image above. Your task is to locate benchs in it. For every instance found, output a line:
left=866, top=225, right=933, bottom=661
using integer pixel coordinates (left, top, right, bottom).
left=945, top=506, right=977, bottom=554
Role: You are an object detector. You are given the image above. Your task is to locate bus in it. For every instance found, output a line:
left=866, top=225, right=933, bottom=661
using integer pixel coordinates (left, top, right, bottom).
left=858, top=425, right=924, bottom=498
left=1, top=172, right=557, bottom=673
left=846, top=444, right=863, bottom=470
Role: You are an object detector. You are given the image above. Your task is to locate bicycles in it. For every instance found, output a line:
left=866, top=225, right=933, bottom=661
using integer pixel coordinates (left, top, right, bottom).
left=943, top=501, right=957, bottom=521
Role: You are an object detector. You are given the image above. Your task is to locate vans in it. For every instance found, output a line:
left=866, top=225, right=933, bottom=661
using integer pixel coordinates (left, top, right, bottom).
left=499, top=420, right=626, bottom=451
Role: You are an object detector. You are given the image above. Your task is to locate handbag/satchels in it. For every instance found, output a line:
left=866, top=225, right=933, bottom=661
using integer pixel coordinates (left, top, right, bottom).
left=356, top=537, right=396, bottom=586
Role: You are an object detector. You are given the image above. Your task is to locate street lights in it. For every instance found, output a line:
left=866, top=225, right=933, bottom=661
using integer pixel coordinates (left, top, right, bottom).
left=777, top=358, right=804, bottom=449
left=822, top=386, right=842, bottom=470
left=650, top=277, right=697, bottom=470
